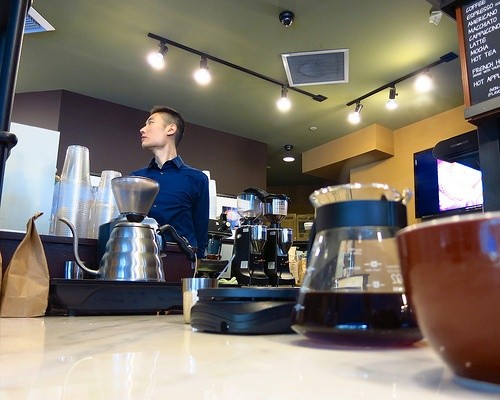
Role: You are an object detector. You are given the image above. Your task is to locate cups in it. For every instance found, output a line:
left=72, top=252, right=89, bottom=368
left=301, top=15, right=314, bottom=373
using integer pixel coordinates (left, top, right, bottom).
left=64, top=261, right=86, bottom=281
left=94, top=170, right=123, bottom=239
left=55, top=144, right=92, bottom=238
left=180, top=277, right=211, bottom=323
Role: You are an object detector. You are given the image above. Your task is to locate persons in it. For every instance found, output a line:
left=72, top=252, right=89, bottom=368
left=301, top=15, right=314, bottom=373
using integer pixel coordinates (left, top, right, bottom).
left=130, top=106, right=210, bottom=269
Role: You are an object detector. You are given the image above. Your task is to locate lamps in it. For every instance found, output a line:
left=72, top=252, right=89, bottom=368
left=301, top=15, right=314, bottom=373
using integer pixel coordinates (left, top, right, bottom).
left=346, top=100, right=363, bottom=126
left=145, top=40, right=169, bottom=72
left=282, top=145, right=295, bottom=162
left=274, top=85, right=292, bottom=112
left=192, top=54, right=213, bottom=87
left=385, top=85, right=399, bottom=111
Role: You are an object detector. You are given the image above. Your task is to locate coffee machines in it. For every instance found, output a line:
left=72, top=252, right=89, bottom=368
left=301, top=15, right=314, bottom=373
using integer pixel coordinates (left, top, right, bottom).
left=219, top=188, right=296, bottom=286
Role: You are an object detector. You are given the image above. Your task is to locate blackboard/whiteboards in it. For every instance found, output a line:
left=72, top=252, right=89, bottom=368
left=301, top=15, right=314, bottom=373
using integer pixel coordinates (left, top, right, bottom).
left=456, top=0, right=500, bottom=108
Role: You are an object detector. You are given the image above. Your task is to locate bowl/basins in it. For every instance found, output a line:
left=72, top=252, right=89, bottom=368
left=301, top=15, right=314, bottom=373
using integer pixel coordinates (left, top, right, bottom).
left=395, top=211, right=500, bottom=394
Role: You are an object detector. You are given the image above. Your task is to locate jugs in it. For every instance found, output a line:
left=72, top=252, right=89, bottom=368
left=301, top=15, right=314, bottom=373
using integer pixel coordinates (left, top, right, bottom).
left=288, top=181, right=425, bottom=349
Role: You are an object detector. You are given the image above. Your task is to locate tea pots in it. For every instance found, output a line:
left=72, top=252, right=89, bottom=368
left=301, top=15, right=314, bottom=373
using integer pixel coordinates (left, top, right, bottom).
left=58, top=213, right=196, bottom=282
